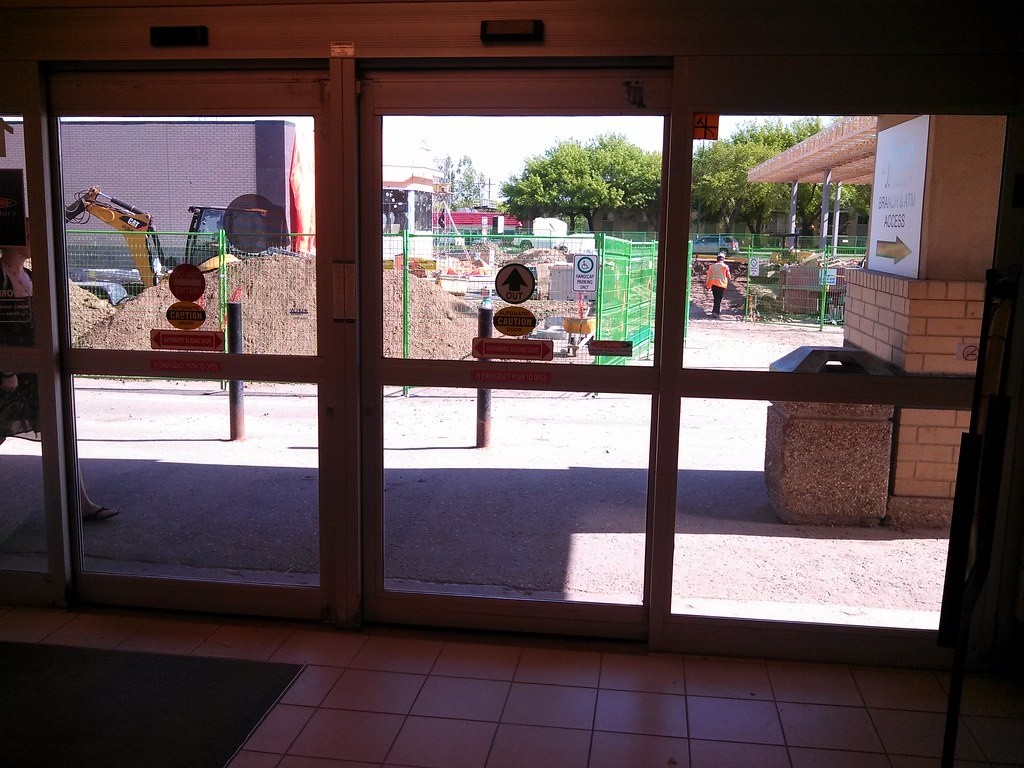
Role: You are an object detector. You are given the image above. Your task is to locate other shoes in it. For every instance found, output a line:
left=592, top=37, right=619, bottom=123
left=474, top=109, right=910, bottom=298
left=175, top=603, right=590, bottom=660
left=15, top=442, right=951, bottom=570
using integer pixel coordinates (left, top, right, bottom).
left=711, top=312, right=719, bottom=318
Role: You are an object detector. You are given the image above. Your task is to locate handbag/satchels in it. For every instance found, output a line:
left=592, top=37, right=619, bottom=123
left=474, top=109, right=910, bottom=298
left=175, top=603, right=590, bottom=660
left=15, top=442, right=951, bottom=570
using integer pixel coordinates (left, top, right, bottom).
left=438, top=218, right=445, bottom=229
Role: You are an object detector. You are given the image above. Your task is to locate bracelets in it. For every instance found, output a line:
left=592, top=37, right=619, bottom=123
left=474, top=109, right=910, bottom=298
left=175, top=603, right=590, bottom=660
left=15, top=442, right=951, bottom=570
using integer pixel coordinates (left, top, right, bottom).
left=3, top=372, right=15, bottom=378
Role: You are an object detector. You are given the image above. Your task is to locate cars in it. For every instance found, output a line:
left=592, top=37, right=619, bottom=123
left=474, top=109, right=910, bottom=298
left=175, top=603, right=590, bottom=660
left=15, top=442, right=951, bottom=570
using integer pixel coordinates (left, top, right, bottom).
left=441, top=232, right=459, bottom=244
left=692, top=235, right=738, bottom=258
left=512, top=234, right=569, bottom=252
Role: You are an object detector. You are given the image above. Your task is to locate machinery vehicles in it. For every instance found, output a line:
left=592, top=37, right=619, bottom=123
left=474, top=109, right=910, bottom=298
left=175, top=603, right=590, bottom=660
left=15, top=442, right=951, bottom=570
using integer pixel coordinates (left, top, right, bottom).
left=63, top=185, right=272, bottom=291
left=770, top=232, right=815, bottom=266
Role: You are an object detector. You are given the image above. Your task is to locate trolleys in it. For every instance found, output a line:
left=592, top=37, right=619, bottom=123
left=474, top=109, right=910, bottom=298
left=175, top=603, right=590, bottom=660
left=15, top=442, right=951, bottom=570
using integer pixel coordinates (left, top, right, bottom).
left=560, top=316, right=596, bottom=357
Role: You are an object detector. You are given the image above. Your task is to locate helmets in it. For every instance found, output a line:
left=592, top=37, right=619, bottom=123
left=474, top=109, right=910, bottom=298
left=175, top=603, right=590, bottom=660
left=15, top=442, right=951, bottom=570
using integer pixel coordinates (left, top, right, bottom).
left=717, top=253, right=725, bottom=258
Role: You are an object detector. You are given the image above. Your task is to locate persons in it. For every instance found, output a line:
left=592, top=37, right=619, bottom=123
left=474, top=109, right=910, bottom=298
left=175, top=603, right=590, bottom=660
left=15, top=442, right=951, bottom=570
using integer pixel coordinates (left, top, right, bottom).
left=0, top=218, right=122, bottom=523
left=705, top=253, right=731, bottom=317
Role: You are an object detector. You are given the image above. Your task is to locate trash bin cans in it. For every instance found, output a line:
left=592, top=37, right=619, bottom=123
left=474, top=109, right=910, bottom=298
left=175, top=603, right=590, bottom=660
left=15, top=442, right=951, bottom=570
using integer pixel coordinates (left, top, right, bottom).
left=764, top=346, right=900, bottom=524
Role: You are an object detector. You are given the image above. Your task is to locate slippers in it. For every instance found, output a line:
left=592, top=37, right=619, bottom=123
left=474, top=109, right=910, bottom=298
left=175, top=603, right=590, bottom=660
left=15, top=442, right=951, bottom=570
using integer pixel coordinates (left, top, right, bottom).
left=82, top=505, right=119, bottom=520
